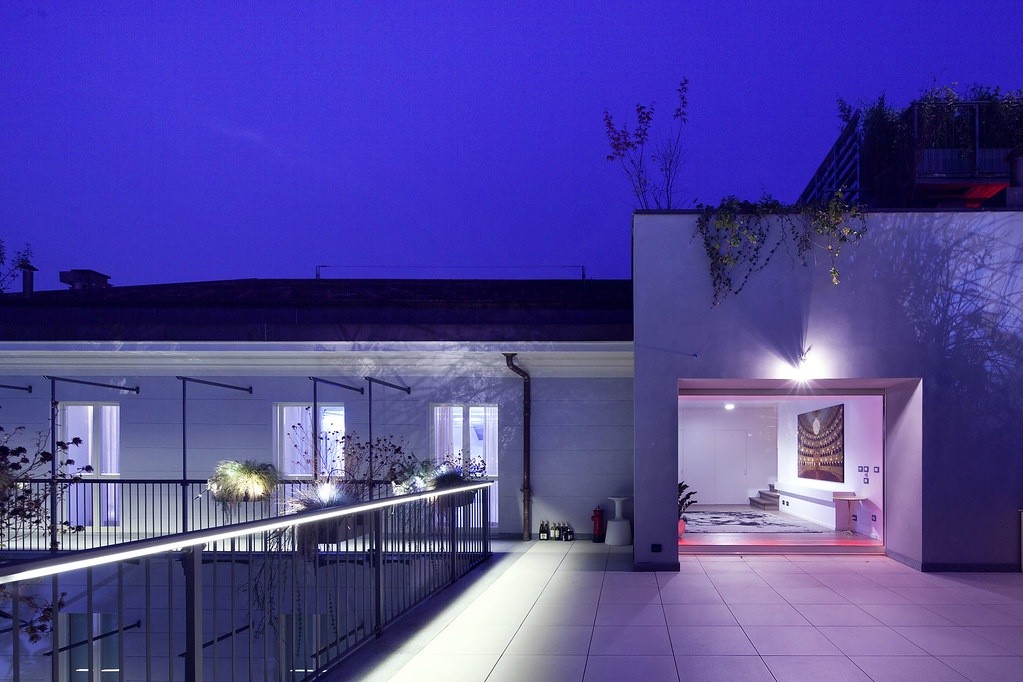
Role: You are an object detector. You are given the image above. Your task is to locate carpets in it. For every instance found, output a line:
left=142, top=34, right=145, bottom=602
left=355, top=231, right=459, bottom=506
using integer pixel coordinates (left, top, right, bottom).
left=682, top=510, right=821, bottom=533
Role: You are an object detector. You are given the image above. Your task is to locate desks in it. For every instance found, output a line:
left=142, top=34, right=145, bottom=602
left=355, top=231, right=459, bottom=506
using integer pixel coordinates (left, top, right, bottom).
left=834, top=496, right=868, bottom=536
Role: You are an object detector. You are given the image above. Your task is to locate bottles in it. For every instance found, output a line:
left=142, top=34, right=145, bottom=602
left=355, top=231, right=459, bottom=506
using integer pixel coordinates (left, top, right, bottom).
left=544, top=520, right=549, bottom=540
left=539, top=520, right=544, bottom=540
left=564, top=521, right=573, bottom=540
left=554, top=522, right=560, bottom=540
left=560, top=523, right=564, bottom=540
left=550, top=522, right=555, bottom=540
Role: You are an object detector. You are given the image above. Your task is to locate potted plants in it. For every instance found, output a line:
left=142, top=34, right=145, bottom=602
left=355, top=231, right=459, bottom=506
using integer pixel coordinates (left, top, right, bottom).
left=678, top=481, right=698, bottom=539
left=209, top=407, right=488, bottom=542
